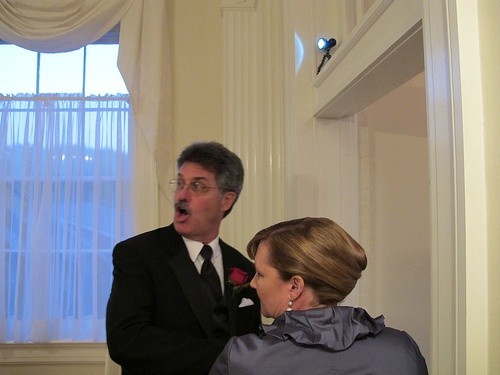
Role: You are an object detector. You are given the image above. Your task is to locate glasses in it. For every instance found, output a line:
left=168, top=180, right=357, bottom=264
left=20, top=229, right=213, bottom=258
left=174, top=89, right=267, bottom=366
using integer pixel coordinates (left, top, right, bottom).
left=168, top=179, right=218, bottom=195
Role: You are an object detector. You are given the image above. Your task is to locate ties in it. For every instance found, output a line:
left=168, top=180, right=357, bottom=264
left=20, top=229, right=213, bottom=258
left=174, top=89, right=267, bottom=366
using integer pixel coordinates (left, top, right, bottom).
left=200, top=245, right=222, bottom=293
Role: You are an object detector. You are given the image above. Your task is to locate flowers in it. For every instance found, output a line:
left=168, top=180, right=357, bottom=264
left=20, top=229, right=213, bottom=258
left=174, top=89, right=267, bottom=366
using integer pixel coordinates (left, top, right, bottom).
left=227, top=267, right=250, bottom=300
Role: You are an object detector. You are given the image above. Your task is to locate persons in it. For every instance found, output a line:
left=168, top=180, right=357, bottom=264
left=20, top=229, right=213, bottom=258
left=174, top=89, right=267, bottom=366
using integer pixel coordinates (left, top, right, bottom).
left=207, top=218, right=429, bottom=375
left=106, top=143, right=263, bottom=375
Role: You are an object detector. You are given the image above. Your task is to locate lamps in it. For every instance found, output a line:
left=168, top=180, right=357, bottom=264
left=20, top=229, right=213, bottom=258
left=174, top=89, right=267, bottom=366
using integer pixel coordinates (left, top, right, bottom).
left=317, top=37, right=337, bottom=74
